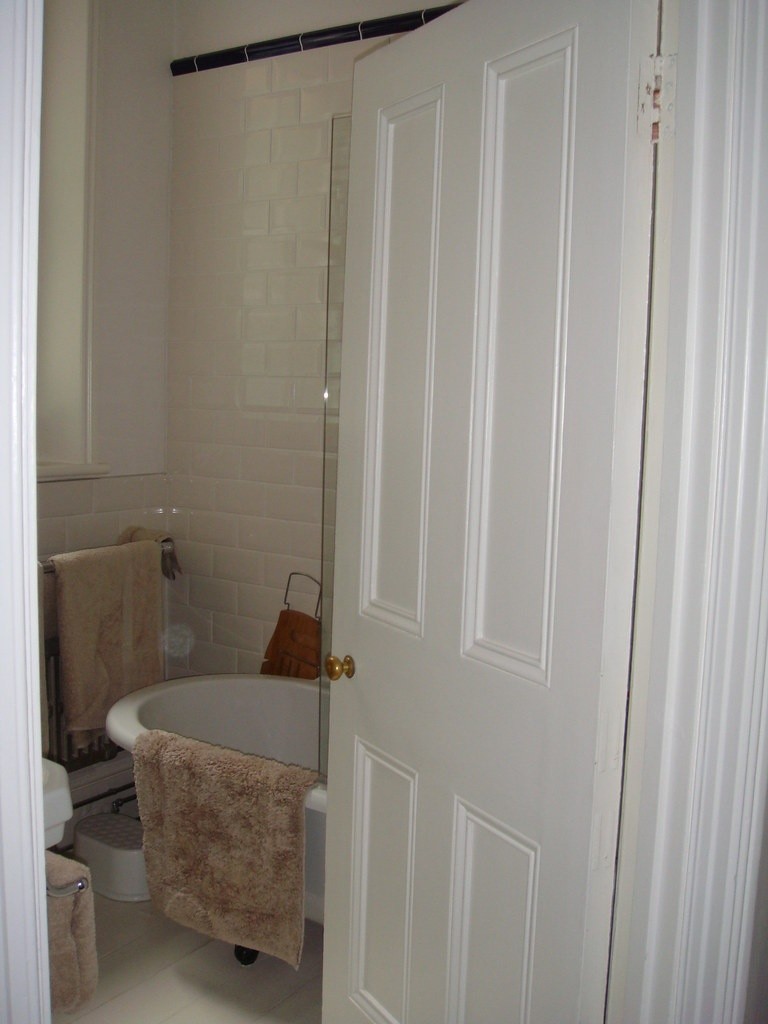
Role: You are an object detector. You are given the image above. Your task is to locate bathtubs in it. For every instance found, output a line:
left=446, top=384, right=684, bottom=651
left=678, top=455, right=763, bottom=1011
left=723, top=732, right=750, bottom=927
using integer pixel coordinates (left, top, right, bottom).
left=107, top=674, right=330, bottom=936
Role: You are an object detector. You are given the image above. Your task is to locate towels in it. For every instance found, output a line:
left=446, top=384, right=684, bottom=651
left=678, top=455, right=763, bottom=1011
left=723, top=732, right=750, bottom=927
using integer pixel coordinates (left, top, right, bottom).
left=49, top=541, right=163, bottom=733
left=38, top=560, right=50, bottom=753
left=132, top=730, right=317, bottom=971
left=115, top=528, right=183, bottom=581
left=45, top=849, right=97, bottom=1010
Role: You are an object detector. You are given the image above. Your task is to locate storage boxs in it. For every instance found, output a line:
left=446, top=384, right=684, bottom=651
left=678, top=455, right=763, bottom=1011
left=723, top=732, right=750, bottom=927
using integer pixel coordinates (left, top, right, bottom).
left=71, top=812, right=151, bottom=902
